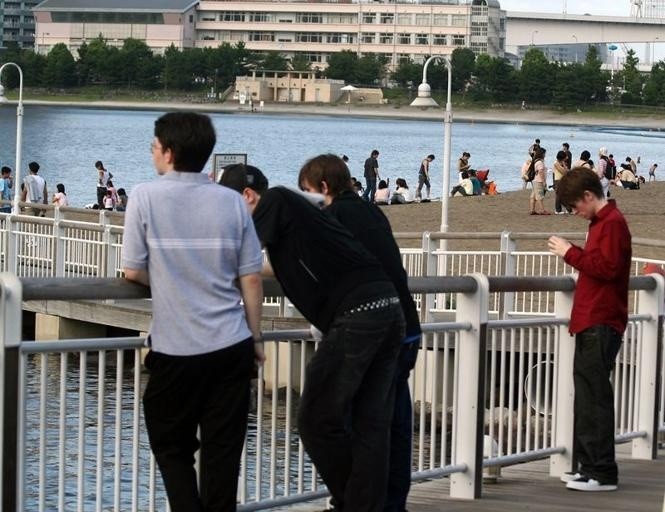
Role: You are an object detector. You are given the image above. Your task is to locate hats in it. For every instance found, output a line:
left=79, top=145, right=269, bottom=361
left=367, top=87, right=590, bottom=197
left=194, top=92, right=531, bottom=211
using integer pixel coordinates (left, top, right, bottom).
left=600, top=147, right=608, bottom=156
left=215, top=164, right=267, bottom=192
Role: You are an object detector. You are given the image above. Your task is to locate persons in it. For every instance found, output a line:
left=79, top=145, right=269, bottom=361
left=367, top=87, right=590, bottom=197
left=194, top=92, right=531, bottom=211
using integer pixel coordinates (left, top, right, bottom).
left=297, top=154, right=422, bottom=510
left=547, top=164, right=634, bottom=491
left=95, top=161, right=127, bottom=211
left=122, top=109, right=269, bottom=512
left=529, top=139, right=658, bottom=214
left=343, top=150, right=412, bottom=204
left=2, top=161, right=68, bottom=217
left=417, top=154, right=435, bottom=200
left=211, top=164, right=406, bottom=512
left=450, top=152, right=482, bottom=196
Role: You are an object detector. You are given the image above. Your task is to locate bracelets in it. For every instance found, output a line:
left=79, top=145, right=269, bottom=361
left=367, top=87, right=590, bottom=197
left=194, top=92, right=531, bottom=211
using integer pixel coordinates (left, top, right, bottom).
left=252, top=332, right=264, bottom=344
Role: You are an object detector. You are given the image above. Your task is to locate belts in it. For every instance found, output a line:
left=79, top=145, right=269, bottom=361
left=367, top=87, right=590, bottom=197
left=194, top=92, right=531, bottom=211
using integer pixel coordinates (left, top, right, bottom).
left=342, top=295, right=401, bottom=317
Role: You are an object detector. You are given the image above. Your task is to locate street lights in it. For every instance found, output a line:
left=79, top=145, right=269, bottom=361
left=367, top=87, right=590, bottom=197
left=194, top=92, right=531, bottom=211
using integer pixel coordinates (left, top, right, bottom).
left=652, top=36, right=659, bottom=67
left=409, top=55, right=454, bottom=308
left=571, top=33, right=578, bottom=63
left=0, top=61, right=24, bottom=215
left=608, top=44, right=617, bottom=89
left=529, top=29, right=539, bottom=46
left=214, top=68, right=218, bottom=94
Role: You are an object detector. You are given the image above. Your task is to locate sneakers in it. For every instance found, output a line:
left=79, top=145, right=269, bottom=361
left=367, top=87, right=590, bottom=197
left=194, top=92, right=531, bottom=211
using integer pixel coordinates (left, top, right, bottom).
left=530, top=210, right=575, bottom=215
left=560, top=471, right=584, bottom=482
left=566, top=476, right=617, bottom=491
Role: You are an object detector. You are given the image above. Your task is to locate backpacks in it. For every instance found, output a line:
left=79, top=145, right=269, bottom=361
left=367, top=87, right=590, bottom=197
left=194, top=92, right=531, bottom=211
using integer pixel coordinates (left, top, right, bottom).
left=603, top=158, right=616, bottom=179
left=523, top=159, right=541, bottom=182
